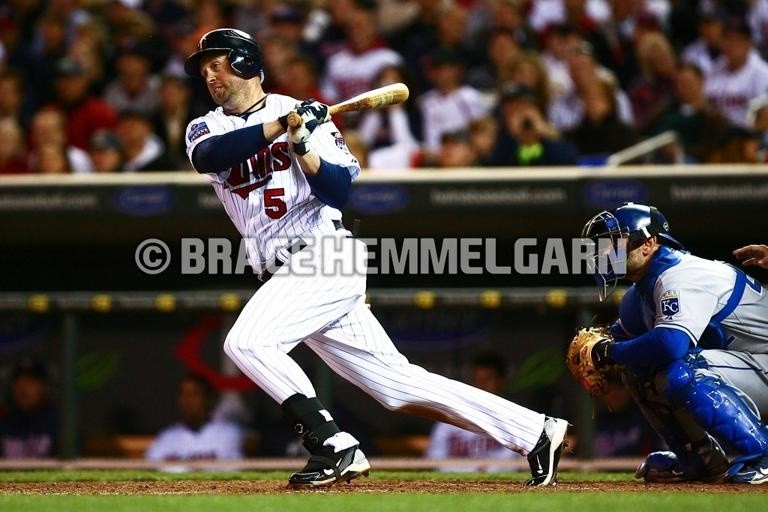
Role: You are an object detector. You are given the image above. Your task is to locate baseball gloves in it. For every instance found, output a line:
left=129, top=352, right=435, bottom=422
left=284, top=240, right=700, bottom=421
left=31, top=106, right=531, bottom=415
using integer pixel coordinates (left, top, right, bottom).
left=568, top=325, right=620, bottom=397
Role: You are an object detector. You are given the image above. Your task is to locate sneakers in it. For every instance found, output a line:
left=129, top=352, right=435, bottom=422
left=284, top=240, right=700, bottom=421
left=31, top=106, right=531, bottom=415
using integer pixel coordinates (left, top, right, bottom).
left=523, top=417, right=569, bottom=486
left=645, top=448, right=727, bottom=484
left=287, top=445, right=370, bottom=489
left=730, top=457, right=768, bottom=485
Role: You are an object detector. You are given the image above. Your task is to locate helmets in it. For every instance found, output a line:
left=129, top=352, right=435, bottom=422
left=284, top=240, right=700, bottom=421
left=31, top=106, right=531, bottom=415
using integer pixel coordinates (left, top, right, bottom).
left=184, top=28, right=269, bottom=85
left=583, top=201, right=682, bottom=287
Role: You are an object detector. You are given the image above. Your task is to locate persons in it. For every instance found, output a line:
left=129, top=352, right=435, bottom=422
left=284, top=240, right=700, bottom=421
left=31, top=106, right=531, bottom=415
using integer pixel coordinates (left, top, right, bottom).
left=183, top=27, right=572, bottom=489
left=565, top=202, right=768, bottom=487
left=729, top=242, right=768, bottom=271
left=2, top=0, right=768, bottom=175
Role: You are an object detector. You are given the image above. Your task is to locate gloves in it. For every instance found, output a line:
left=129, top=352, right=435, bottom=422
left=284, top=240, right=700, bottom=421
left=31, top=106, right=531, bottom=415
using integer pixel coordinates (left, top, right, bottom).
left=278, top=99, right=331, bottom=155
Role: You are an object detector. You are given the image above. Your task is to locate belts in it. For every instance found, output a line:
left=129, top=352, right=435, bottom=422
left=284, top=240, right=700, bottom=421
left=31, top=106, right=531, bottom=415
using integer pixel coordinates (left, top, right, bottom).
left=258, top=220, right=345, bottom=280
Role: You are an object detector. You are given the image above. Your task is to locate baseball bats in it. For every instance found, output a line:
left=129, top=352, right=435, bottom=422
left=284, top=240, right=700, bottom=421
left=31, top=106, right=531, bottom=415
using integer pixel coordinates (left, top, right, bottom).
left=287, top=82, right=409, bottom=127
